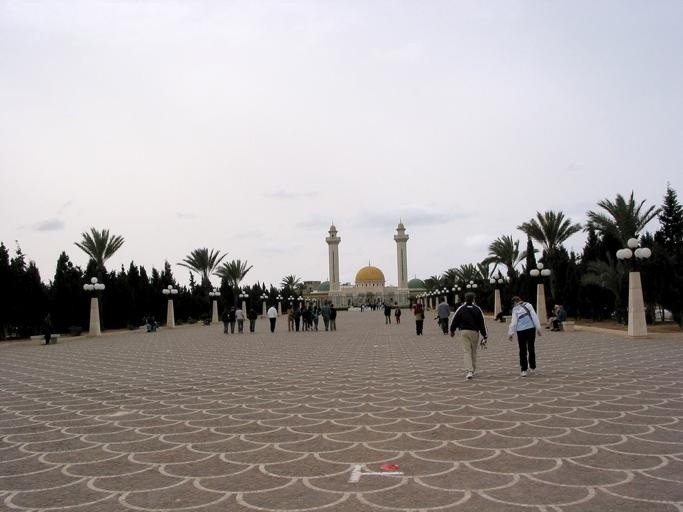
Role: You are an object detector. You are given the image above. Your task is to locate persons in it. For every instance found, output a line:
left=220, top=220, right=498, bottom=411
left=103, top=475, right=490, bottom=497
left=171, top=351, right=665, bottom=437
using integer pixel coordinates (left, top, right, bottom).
left=545, top=304, right=567, bottom=331
left=414, top=303, right=425, bottom=336
left=44, top=312, right=51, bottom=344
left=508, top=296, right=543, bottom=376
left=450, top=292, right=488, bottom=379
left=495, top=303, right=509, bottom=322
left=221, top=302, right=336, bottom=334
left=436, top=298, right=466, bottom=335
left=361, top=301, right=401, bottom=324
left=143, top=316, right=159, bottom=332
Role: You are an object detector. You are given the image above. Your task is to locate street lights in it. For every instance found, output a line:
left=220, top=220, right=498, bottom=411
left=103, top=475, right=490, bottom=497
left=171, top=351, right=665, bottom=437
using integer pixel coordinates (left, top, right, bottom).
left=528, top=261, right=552, bottom=324
left=238, top=290, right=249, bottom=321
left=159, top=284, right=179, bottom=328
left=413, top=286, right=450, bottom=311
left=207, top=288, right=221, bottom=326
left=80, top=276, right=106, bottom=336
left=259, top=292, right=269, bottom=316
left=487, top=273, right=506, bottom=320
left=613, top=237, right=652, bottom=337
left=451, top=283, right=461, bottom=305
left=274, top=293, right=284, bottom=316
left=464, top=279, right=477, bottom=292
left=287, top=294, right=317, bottom=311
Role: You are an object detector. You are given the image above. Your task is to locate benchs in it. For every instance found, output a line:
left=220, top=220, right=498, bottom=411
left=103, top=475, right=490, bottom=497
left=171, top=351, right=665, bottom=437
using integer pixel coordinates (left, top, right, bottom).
left=557, top=320, right=576, bottom=333
left=29, top=333, right=61, bottom=344
left=501, top=315, right=511, bottom=323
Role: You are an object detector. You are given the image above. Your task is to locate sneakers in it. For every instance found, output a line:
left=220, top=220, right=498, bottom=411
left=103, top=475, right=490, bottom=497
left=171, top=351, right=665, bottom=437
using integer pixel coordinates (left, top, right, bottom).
left=466, top=372, right=473, bottom=379
left=521, top=370, right=527, bottom=377
left=528, top=367, right=535, bottom=373
